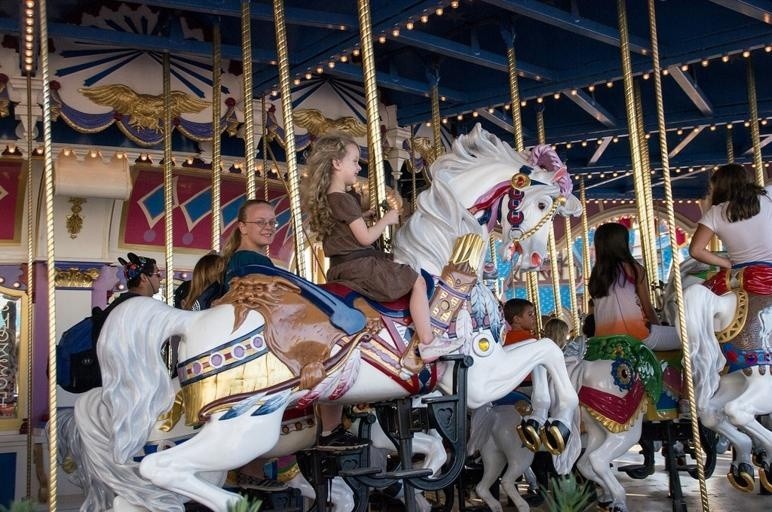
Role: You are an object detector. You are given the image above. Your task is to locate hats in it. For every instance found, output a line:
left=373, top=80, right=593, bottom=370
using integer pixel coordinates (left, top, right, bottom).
left=117, top=252, right=155, bottom=281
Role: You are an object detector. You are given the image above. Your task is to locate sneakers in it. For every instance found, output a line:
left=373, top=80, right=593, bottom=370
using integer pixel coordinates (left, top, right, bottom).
left=417, top=334, right=466, bottom=364
left=318, top=423, right=373, bottom=451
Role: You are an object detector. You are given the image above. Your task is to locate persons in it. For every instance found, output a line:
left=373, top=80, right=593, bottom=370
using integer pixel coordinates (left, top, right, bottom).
left=178, top=250, right=227, bottom=313
left=219, top=198, right=279, bottom=294
left=582, top=294, right=600, bottom=340
left=685, top=162, right=772, bottom=271
left=586, top=220, right=697, bottom=420
left=88, top=251, right=163, bottom=343
left=543, top=314, right=569, bottom=354
left=504, top=298, right=541, bottom=392
left=298, top=133, right=467, bottom=367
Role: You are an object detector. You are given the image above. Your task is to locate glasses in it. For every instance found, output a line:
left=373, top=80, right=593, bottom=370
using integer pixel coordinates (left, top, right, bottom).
left=243, top=218, right=280, bottom=228
left=150, top=270, right=163, bottom=277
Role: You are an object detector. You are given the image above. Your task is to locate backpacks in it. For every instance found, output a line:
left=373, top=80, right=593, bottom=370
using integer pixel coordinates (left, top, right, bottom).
left=47, top=294, right=140, bottom=394
